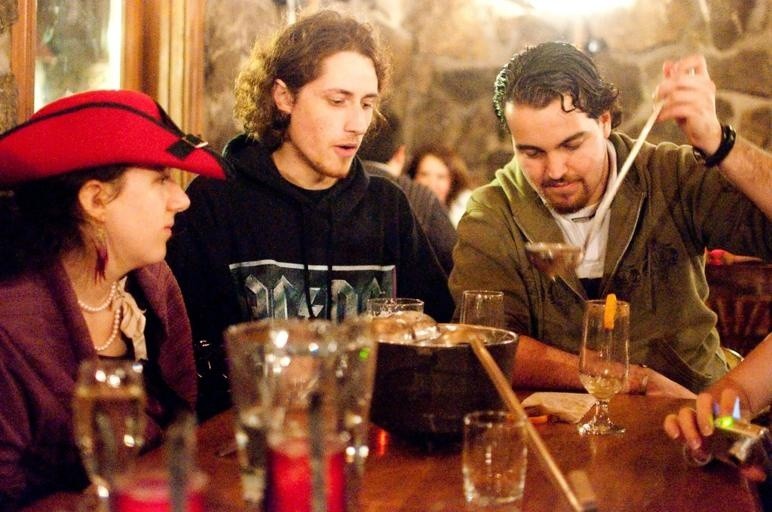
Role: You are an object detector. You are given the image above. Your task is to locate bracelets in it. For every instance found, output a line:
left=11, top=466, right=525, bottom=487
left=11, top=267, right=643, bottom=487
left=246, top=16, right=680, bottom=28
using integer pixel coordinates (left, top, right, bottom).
left=637, top=361, right=650, bottom=397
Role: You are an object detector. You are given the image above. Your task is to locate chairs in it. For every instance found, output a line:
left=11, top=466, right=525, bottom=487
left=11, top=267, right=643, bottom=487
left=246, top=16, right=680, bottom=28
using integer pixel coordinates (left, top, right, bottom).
left=706, top=258, right=772, bottom=360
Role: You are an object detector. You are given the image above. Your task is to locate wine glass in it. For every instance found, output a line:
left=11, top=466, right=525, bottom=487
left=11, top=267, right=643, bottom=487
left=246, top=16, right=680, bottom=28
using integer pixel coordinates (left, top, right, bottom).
left=73, top=361, right=146, bottom=512
left=579, top=299, right=631, bottom=436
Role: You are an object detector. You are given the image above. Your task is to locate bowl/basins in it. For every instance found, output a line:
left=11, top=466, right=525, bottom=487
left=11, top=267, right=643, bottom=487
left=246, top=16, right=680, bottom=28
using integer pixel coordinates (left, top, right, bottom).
left=368, top=324, right=518, bottom=451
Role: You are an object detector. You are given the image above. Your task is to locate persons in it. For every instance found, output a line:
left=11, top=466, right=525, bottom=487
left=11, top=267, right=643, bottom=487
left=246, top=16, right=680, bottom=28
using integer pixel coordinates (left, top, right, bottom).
left=357, top=108, right=457, bottom=256
left=167, top=8, right=457, bottom=425
left=445, top=39, right=772, bottom=394
left=407, top=142, right=475, bottom=231
left=662, top=325, right=772, bottom=483
left=0, top=87, right=227, bottom=509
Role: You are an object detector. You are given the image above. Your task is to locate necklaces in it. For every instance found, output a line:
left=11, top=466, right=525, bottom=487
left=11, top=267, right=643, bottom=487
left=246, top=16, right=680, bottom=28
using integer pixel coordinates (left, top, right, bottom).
left=77, top=278, right=119, bottom=353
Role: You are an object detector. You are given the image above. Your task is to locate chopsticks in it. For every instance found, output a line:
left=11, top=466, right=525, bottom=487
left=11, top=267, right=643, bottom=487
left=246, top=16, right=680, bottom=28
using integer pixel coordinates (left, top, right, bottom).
left=468, top=333, right=584, bottom=512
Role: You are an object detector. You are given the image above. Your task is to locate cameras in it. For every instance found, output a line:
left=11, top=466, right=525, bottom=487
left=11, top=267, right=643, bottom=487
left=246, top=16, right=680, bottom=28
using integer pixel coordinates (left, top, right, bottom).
left=694, top=416, right=772, bottom=481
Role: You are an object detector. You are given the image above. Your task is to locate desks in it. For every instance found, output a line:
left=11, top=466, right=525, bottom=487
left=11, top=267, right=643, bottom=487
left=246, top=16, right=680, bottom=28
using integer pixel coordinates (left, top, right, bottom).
left=24, top=388, right=761, bottom=512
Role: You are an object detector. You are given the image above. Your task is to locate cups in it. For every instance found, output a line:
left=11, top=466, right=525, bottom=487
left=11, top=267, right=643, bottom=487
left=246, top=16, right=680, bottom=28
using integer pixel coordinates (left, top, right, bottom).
left=223, top=319, right=377, bottom=512
left=463, top=411, right=528, bottom=508
left=368, top=290, right=505, bottom=329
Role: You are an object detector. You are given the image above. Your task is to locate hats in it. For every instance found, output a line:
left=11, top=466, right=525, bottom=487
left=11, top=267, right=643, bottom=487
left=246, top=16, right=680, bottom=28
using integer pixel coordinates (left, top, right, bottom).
left=0, top=90, right=236, bottom=185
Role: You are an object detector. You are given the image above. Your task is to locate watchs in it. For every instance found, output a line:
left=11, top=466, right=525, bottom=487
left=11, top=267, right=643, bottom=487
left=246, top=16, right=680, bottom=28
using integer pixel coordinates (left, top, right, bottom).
left=688, top=119, right=742, bottom=169
